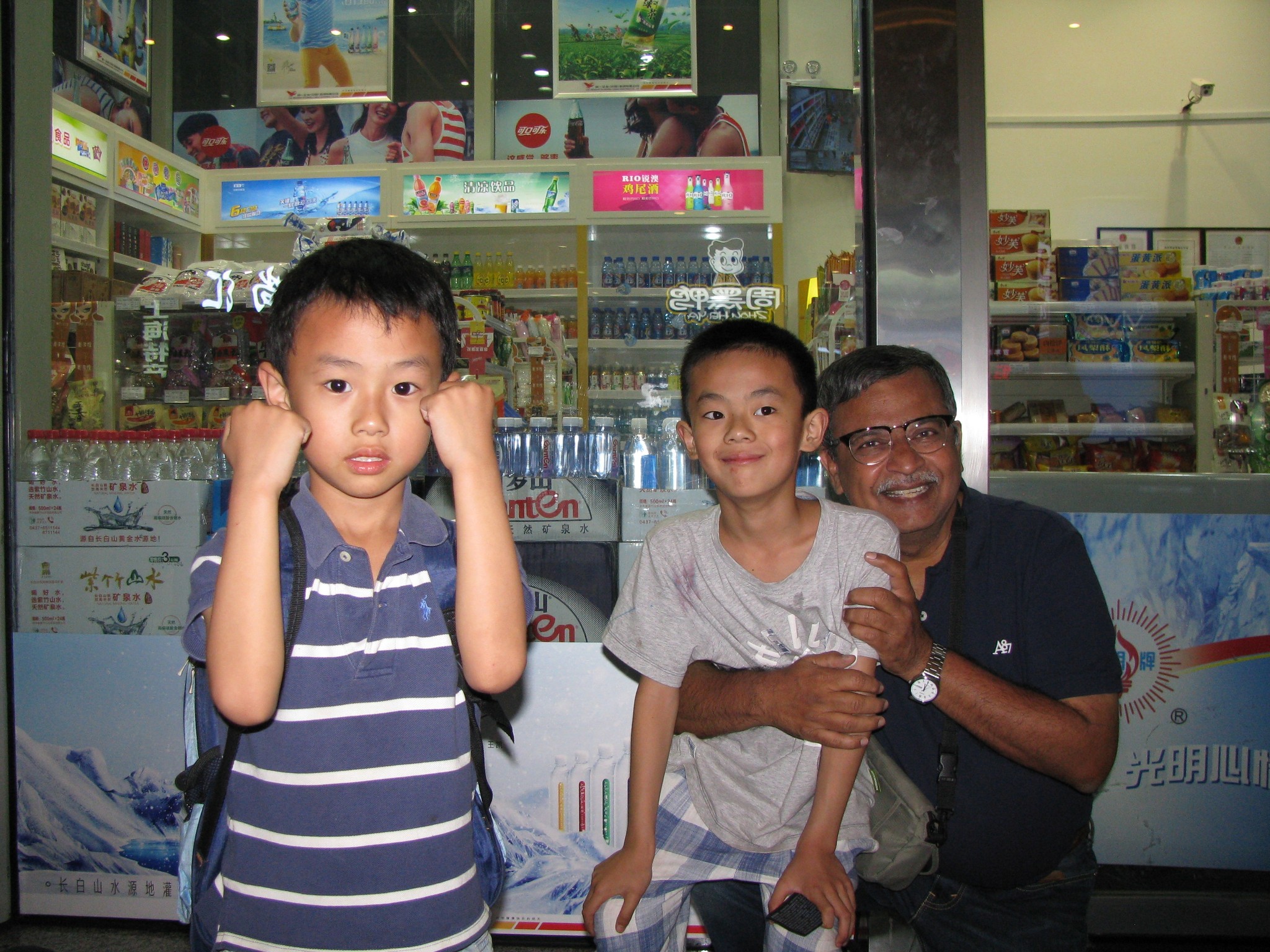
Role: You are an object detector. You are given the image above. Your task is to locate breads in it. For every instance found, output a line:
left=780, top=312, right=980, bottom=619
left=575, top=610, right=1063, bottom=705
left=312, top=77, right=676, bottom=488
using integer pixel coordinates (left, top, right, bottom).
left=1002, top=331, right=1040, bottom=363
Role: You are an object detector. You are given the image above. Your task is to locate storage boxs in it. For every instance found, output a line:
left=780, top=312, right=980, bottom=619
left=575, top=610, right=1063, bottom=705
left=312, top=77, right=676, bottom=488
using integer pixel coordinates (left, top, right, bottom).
left=990, top=207, right=1193, bottom=364
left=52, top=271, right=135, bottom=303
left=18, top=477, right=837, bottom=643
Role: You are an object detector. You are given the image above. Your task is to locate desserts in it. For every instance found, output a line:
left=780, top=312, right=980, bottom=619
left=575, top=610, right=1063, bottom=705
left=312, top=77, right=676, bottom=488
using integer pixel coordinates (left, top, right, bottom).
left=1021, top=233, right=1046, bottom=301
left=1124, top=259, right=1189, bottom=302
left=1083, top=248, right=1121, bottom=303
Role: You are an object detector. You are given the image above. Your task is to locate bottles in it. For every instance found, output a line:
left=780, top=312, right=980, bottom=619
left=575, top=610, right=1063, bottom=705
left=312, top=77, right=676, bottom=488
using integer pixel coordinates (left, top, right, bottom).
left=685, top=172, right=734, bottom=210
left=19, top=223, right=832, bottom=490
left=284, top=0, right=299, bottom=17
left=343, top=145, right=353, bottom=165
left=542, top=177, right=558, bottom=212
left=427, top=176, right=442, bottom=213
left=337, top=201, right=369, bottom=216
left=347, top=24, right=378, bottom=54
left=449, top=199, right=474, bottom=214
left=568, top=99, right=585, bottom=159
left=280, top=138, right=294, bottom=167
left=412, top=175, right=430, bottom=211
left=293, top=181, right=306, bottom=213
left=621, top=0, right=669, bottom=53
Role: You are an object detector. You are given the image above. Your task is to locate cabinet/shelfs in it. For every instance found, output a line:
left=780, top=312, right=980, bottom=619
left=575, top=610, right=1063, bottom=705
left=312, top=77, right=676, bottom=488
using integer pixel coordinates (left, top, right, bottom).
left=991, top=293, right=1216, bottom=474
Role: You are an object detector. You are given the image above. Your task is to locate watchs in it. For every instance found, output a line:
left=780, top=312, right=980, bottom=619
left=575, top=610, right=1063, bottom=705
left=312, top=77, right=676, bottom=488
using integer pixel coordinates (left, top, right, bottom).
left=908, top=642, right=948, bottom=705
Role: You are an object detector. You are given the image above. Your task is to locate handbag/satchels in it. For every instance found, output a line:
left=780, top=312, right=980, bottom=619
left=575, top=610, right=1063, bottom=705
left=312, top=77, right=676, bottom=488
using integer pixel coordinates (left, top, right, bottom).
left=855, top=735, right=948, bottom=890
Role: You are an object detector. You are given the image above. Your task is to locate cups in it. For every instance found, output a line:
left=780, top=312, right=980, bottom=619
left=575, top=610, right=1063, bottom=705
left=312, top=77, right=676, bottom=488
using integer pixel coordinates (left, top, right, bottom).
left=495, top=202, right=508, bottom=214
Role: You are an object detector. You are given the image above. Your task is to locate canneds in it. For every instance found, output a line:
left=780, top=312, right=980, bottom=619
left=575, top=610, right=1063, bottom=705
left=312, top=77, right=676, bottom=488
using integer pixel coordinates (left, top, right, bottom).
left=511, top=199, right=519, bottom=213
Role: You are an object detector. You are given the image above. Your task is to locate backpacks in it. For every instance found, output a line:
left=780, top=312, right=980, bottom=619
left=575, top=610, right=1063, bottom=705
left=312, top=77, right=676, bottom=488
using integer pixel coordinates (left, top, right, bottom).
left=174, top=506, right=515, bottom=924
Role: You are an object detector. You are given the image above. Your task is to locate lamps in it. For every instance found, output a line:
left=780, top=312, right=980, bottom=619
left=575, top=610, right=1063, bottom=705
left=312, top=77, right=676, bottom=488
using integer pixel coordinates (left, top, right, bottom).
left=779, top=61, right=822, bottom=101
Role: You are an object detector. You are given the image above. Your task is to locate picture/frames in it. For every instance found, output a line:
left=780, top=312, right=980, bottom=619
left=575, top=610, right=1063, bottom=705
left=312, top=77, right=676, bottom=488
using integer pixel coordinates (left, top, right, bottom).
left=552, top=0, right=698, bottom=99
left=75, top=0, right=153, bottom=97
left=257, top=0, right=395, bottom=108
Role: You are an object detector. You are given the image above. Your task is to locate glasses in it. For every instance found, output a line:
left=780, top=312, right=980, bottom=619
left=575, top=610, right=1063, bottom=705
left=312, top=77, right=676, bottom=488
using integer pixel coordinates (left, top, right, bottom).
left=834, top=415, right=955, bottom=464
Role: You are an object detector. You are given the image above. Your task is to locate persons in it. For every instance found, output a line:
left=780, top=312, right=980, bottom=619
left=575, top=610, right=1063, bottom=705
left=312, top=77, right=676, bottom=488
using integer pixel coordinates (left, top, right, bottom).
left=564, top=96, right=750, bottom=158
left=52, top=74, right=150, bottom=141
left=326, top=101, right=467, bottom=165
left=177, top=104, right=345, bottom=170
left=181, top=239, right=535, bottom=952
left=582, top=317, right=1122, bottom=952
left=282, top=0, right=354, bottom=88
